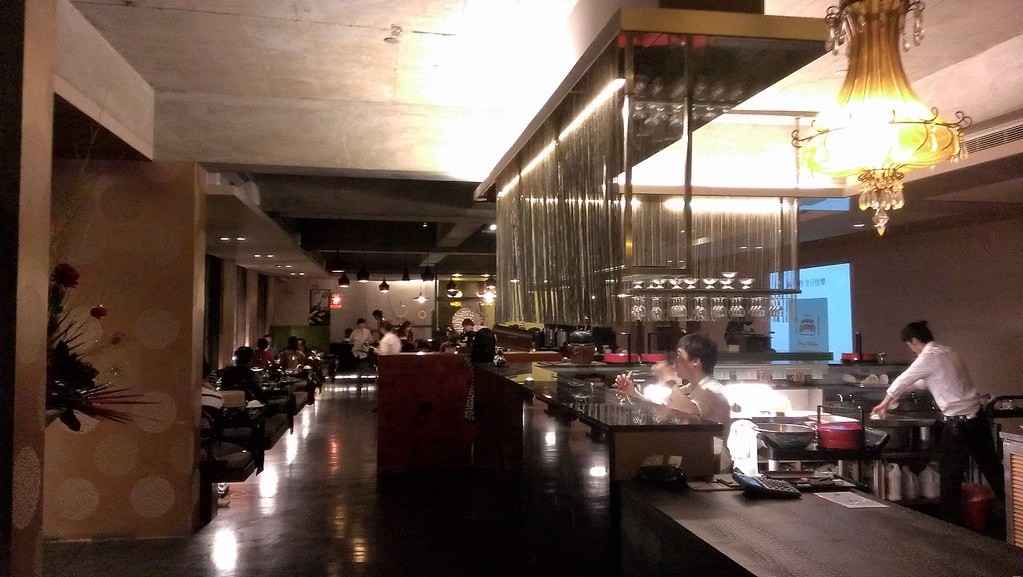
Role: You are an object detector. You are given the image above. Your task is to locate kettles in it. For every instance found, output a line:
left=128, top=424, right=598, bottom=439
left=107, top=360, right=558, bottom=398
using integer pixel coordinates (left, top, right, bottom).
left=569, top=323, right=593, bottom=346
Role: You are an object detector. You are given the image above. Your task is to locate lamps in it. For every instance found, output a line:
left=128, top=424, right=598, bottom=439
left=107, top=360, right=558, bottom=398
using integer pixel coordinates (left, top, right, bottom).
left=791, top=1, right=973, bottom=237
left=324, top=223, right=496, bottom=304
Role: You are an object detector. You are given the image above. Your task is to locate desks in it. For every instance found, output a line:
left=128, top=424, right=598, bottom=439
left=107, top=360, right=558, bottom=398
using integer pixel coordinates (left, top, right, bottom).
left=298, top=358, right=324, bottom=391
left=255, top=380, right=298, bottom=433
left=209, top=406, right=267, bottom=472
left=304, top=352, right=336, bottom=386
left=281, top=366, right=316, bottom=405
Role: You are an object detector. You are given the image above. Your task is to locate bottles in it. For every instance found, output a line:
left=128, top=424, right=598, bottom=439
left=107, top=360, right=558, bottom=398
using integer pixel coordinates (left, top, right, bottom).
left=918, top=465, right=943, bottom=498
left=881, top=458, right=904, bottom=501
left=899, top=465, right=920, bottom=501
left=864, top=460, right=885, bottom=500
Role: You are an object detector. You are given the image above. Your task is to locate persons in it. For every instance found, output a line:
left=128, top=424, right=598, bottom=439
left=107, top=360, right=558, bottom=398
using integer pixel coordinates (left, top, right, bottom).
left=431, top=326, right=458, bottom=353
left=263, top=335, right=279, bottom=359
left=465, top=328, right=506, bottom=423
left=202, top=353, right=216, bottom=387
left=252, top=338, right=277, bottom=368
left=373, top=310, right=386, bottom=340
left=462, top=318, right=485, bottom=332
left=348, top=319, right=371, bottom=359
left=216, top=346, right=263, bottom=401
left=396, top=321, right=416, bottom=342
left=298, top=338, right=314, bottom=356
left=615, top=332, right=734, bottom=474
left=373, top=321, right=402, bottom=355
left=870, top=322, right=1005, bottom=523
left=280, top=336, right=305, bottom=368
left=643, top=361, right=682, bottom=404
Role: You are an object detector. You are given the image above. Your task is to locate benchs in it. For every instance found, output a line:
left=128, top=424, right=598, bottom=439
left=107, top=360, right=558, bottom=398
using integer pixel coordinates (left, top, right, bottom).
left=198, top=350, right=337, bottom=481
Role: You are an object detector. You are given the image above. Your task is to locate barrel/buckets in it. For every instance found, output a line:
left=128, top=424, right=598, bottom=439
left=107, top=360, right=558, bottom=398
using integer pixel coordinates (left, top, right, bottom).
left=960, top=483, right=991, bottom=530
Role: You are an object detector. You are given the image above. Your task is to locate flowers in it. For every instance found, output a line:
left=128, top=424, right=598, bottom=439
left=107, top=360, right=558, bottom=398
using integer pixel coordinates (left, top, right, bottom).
left=46, top=263, right=166, bottom=433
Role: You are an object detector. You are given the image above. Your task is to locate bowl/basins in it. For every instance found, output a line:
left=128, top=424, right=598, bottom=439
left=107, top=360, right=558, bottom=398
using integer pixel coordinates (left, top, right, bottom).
left=752, top=423, right=816, bottom=451
left=843, top=373, right=890, bottom=386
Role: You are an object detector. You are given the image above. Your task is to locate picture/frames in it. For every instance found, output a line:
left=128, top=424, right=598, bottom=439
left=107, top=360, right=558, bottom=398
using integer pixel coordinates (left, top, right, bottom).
left=309, top=288, right=330, bottom=325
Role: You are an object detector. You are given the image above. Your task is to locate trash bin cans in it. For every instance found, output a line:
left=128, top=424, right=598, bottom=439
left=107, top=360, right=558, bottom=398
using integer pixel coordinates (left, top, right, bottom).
left=962, top=484, right=995, bottom=537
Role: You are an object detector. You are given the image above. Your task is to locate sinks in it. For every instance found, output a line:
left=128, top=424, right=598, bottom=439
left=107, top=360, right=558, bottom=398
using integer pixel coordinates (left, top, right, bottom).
left=862, top=409, right=943, bottom=428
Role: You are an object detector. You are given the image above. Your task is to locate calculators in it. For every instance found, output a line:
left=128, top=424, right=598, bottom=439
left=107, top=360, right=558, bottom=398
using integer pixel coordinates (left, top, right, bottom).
left=732, top=472, right=802, bottom=500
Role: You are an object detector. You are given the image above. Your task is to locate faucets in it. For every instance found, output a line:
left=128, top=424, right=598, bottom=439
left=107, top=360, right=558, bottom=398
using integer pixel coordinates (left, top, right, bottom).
left=906, top=392, right=918, bottom=406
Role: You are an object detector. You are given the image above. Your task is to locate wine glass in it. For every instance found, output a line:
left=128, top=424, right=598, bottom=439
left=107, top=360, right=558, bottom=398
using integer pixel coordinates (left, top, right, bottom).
left=631, top=293, right=781, bottom=322
